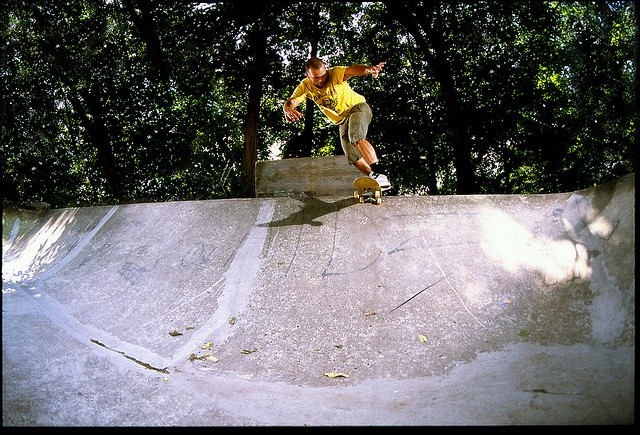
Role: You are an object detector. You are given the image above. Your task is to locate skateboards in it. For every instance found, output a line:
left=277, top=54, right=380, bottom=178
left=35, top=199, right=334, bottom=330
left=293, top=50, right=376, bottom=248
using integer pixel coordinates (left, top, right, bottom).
left=352, top=177, right=382, bottom=205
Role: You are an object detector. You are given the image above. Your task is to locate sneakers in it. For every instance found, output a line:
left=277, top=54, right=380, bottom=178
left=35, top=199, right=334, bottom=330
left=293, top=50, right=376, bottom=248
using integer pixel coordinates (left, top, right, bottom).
left=370, top=174, right=390, bottom=191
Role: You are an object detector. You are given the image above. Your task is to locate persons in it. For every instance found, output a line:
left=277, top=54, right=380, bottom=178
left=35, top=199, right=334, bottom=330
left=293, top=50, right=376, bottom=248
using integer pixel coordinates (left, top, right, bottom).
left=282, top=57, right=392, bottom=188
